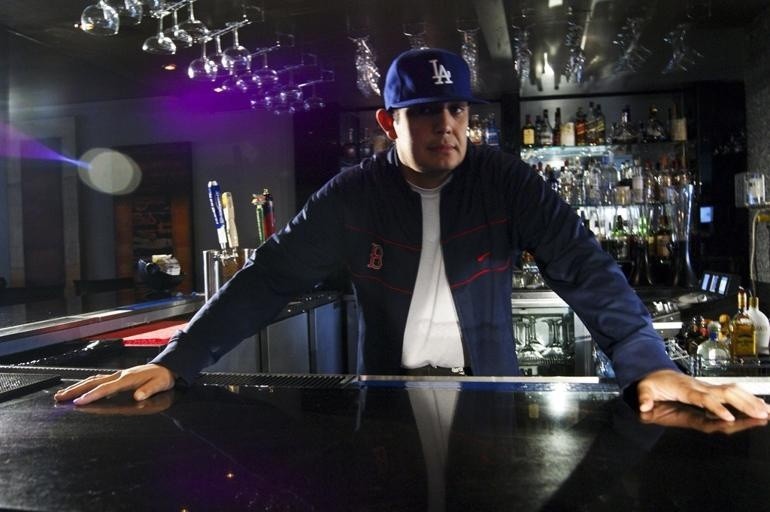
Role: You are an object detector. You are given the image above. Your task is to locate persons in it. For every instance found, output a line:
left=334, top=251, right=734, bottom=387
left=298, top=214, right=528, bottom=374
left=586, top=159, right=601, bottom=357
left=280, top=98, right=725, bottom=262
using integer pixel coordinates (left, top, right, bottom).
left=53, top=47, right=769, bottom=424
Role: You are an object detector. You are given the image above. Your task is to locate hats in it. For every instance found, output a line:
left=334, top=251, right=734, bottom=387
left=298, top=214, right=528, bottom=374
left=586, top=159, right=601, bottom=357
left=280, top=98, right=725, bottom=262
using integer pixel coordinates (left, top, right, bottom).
left=383, top=48, right=490, bottom=112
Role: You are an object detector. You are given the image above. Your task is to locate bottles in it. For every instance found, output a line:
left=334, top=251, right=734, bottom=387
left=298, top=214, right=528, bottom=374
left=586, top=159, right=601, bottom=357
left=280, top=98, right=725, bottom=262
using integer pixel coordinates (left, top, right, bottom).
left=519, top=101, right=688, bottom=263
left=470, top=111, right=501, bottom=149
left=697, top=322, right=732, bottom=383
left=618, top=184, right=703, bottom=290
left=745, top=295, right=770, bottom=358
left=729, top=289, right=757, bottom=366
left=338, top=119, right=392, bottom=167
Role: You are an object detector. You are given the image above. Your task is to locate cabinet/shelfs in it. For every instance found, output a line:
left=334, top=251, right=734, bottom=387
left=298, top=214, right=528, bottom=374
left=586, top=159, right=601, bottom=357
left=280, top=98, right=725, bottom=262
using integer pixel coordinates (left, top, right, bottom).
left=290, top=79, right=748, bottom=367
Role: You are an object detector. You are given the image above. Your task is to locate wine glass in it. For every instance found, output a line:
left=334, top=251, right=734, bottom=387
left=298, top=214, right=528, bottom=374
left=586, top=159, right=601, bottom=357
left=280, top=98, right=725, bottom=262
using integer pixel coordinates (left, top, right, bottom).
left=77, top=0, right=329, bottom=116
left=351, top=1, right=713, bottom=98
left=505, top=315, right=569, bottom=366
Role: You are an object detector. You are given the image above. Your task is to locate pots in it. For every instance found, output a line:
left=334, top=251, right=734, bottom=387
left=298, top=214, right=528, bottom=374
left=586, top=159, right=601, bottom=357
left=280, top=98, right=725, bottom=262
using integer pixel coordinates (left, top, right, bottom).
left=137, top=256, right=185, bottom=290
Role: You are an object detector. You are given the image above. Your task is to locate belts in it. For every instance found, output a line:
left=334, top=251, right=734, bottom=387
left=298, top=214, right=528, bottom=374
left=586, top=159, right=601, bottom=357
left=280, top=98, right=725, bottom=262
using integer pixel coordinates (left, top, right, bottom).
left=404, top=367, right=467, bottom=376
